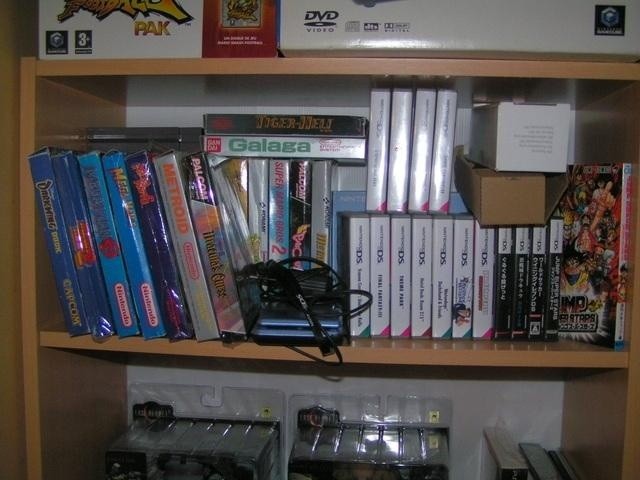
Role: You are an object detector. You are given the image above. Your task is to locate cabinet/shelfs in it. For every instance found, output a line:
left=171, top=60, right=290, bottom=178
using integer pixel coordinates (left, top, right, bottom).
left=19, top=57, right=636, bottom=477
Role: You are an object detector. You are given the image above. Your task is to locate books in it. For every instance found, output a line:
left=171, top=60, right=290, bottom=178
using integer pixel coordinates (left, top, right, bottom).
left=429, top=90, right=457, bottom=217
left=366, top=90, right=391, bottom=216
left=408, top=89, right=437, bottom=216
left=203, top=113, right=368, bottom=137
left=386, top=90, right=416, bottom=214
left=480, top=427, right=577, bottom=479
left=29, top=136, right=631, bottom=351
left=106, top=420, right=281, bottom=479
left=287, top=423, right=450, bottom=479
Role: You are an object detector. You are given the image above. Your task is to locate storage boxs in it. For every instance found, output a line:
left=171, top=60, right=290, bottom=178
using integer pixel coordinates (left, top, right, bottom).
left=454, top=98, right=576, bottom=227
